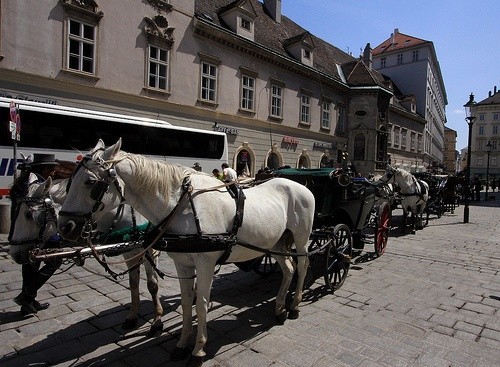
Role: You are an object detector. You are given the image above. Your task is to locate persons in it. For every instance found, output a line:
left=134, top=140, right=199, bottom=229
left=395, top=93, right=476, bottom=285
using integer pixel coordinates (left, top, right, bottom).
left=240, top=165, right=305, bottom=179
left=221, top=163, right=237, bottom=182
left=212, top=169, right=224, bottom=181
left=8, top=154, right=63, bottom=314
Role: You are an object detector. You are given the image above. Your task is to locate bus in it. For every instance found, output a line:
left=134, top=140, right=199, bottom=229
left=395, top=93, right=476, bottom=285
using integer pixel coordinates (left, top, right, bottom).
left=0, top=96, right=229, bottom=200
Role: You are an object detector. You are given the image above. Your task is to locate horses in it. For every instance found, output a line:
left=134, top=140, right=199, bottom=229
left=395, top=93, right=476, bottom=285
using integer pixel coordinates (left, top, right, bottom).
left=370, top=163, right=429, bottom=236
left=10, top=137, right=316, bottom=366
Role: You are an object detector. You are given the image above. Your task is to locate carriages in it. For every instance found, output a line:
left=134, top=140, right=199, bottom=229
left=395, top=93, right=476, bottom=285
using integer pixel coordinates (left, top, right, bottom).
left=8, top=167, right=166, bottom=338
left=59, top=136, right=391, bottom=364
left=366, top=171, right=401, bottom=210
left=380, top=163, right=440, bottom=234
left=428, top=163, right=465, bottom=214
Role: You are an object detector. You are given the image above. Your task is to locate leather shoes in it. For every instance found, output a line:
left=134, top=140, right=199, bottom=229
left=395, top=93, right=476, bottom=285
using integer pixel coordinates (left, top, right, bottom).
left=14, top=292, right=38, bottom=313
left=21, top=300, right=50, bottom=314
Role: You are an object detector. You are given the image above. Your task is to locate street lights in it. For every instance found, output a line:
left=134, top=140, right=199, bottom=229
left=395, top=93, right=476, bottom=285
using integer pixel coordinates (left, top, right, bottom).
left=485, top=138, right=494, bottom=206
left=463, top=93, right=481, bottom=225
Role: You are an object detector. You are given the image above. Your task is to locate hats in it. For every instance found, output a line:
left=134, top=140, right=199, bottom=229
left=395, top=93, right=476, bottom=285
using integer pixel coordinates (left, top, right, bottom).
left=28, top=153, right=59, bottom=166
